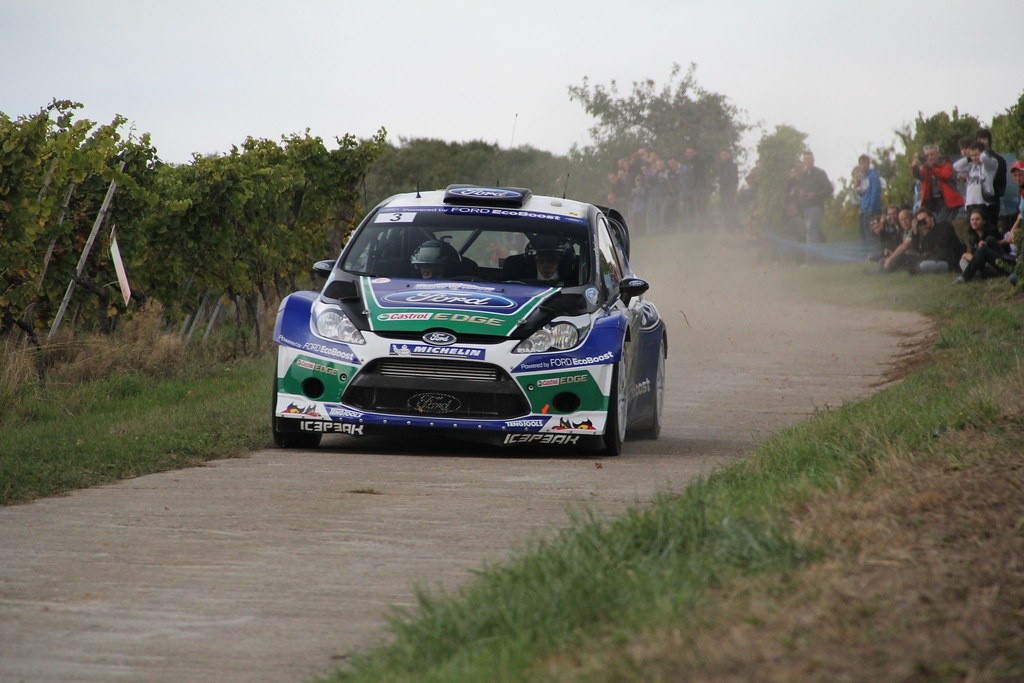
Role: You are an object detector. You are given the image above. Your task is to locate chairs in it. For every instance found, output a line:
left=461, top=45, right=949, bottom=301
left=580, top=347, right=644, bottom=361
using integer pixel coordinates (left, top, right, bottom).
left=502, top=254, right=579, bottom=285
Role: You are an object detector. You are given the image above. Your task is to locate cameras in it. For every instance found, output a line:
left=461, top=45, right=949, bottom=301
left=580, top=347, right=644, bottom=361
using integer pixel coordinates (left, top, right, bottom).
left=916, top=220, right=926, bottom=226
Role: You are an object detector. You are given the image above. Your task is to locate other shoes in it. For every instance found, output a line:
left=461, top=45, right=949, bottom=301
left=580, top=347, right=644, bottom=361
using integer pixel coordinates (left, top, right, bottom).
left=909, top=264, right=916, bottom=276
left=950, top=278, right=963, bottom=286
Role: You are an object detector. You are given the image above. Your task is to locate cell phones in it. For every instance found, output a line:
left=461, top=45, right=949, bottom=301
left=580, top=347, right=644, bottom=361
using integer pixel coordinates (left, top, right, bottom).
left=917, top=153, right=928, bottom=163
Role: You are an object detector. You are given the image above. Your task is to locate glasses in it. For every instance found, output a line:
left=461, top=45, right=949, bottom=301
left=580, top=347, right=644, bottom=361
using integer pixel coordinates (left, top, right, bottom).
left=870, top=220, right=878, bottom=225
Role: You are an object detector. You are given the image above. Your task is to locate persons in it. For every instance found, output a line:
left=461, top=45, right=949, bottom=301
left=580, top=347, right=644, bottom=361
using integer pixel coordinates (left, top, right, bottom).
left=843, top=128, right=1024, bottom=293
left=607, top=147, right=709, bottom=233
left=717, top=150, right=739, bottom=226
left=780, top=150, right=834, bottom=244
left=524, top=233, right=580, bottom=286
left=409, top=239, right=462, bottom=281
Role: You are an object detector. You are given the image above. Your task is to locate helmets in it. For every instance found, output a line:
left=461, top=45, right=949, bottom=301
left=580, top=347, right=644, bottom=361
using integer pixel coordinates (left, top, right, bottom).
left=409, top=240, right=465, bottom=284
left=524, top=232, right=578, bottom=287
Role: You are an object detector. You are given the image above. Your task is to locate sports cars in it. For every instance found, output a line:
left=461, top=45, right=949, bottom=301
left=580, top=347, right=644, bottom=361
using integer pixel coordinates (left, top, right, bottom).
left=271, top=180, right=668, bottom=457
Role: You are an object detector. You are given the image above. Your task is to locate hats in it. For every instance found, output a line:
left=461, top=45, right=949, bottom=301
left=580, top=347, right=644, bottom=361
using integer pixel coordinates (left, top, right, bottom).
left=1010, top=161, right=1024, bottom=174
